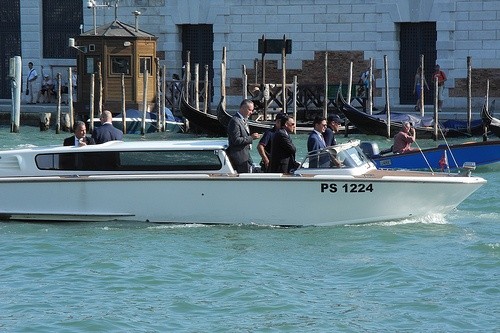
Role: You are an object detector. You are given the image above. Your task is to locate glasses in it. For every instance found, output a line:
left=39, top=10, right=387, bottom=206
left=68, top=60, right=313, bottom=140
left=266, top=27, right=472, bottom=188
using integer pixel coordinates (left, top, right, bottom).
left=287, top=122, right=295, bottom=127
left=320, top=123, right=327, bottom=129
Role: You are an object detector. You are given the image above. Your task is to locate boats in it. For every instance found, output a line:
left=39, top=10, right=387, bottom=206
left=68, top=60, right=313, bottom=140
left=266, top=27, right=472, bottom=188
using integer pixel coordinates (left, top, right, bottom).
left=178, top=86, right=387, bottom=136
left=336, top=80, right=500, bottom=137
left=0, top=134, right=500, bottom=228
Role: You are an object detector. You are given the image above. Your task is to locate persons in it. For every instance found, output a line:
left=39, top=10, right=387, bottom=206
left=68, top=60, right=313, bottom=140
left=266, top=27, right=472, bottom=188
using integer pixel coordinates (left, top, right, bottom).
left=269, top=114, right=302, bottom=173
left=413, top=66, right=429, bottom=110
left=308, top=115, right=342, bottom=168
left=228, top=99, right=262, bottom=173
left=172, top=74, right=180, bottom=81
left=92, top=110, right=123, bottom=144
left=64, top=121, right=96, bottom=146
left=37, top=69, right=78, bottom=104
left=258, top=113, right=288, bottom=172
left=432, top=65, right=448, bottom=112
left=26, top=62, right=39, bottom=104
left=322, top=115, right=344, bottom=146
left=360, top=66, right=378, bottom=111
left=394, top=122, right=419, bottom=153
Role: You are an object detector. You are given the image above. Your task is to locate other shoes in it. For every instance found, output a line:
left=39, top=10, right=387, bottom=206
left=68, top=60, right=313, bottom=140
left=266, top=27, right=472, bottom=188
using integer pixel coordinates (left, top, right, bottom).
left=438, top=108, right=441, bottom=112
left=414, top=106, right=420, bottom=111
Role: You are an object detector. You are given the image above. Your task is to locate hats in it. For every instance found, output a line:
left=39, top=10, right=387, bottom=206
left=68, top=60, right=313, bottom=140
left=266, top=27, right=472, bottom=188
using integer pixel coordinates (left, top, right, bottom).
left=274, top=112, right=287, bottom=121
left=327, top=115, right=344, bottom=123
